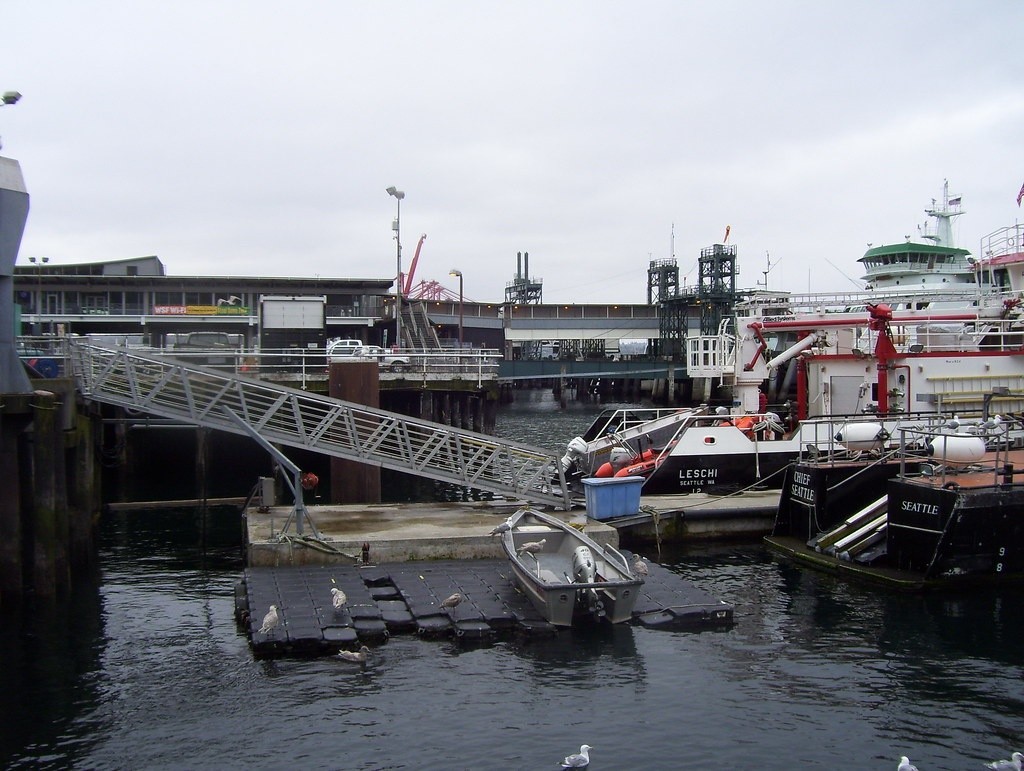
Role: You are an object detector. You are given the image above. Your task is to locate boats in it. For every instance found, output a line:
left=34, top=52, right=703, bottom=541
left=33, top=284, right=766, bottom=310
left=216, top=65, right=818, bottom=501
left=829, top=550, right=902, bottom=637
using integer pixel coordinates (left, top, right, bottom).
left=513, top=176, right=1024, bottom=599
left=498, top=504, right=646, bottom=629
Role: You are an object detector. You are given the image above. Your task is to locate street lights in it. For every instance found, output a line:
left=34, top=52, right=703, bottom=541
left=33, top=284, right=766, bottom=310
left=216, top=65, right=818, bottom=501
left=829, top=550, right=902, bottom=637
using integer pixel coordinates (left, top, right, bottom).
left=448, top=269, right=463, bottom=371
left=29, top=257, right=49, bottom=313
left=385, top=185, right=405, bottom=348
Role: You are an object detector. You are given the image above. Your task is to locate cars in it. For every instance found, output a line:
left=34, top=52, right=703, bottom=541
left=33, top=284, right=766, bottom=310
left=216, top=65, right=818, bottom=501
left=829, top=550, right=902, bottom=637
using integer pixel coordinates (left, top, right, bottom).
left=328, top=338, right=412, bottom=373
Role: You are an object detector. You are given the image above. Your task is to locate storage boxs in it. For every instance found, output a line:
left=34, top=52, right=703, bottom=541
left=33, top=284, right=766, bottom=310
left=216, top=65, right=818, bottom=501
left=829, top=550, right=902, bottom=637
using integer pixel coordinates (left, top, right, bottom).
left=581, top=475, right=645, bottom=519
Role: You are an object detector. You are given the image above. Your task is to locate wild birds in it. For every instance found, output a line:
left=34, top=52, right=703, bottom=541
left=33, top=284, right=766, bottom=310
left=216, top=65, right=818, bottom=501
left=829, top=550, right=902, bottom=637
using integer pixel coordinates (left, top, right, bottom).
left=947, top=415, right=960, bottom=429
left=258, top=604, right=281, bottom=641
left=338, top=645, right=372, bottom=662
left=516, top=538, right=547, bottom=558
left=982, top=752, right=1024, bottom=771
left=897, top=756, right=918, bottom=771
left=330, top=588, right=346, bottom=613
left=978, top=414, right=1001, bottom=429
left=629, top=554, right=648, bottom=580
left=438, top=591, right=465, bottom=615
left=553, top=743, right=594, bottom=770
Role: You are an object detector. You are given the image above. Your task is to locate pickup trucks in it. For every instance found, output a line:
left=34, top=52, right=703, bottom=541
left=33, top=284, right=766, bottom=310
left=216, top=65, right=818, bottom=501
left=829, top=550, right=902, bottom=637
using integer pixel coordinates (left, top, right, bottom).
left=174, top=331, right=238, bottom=375
